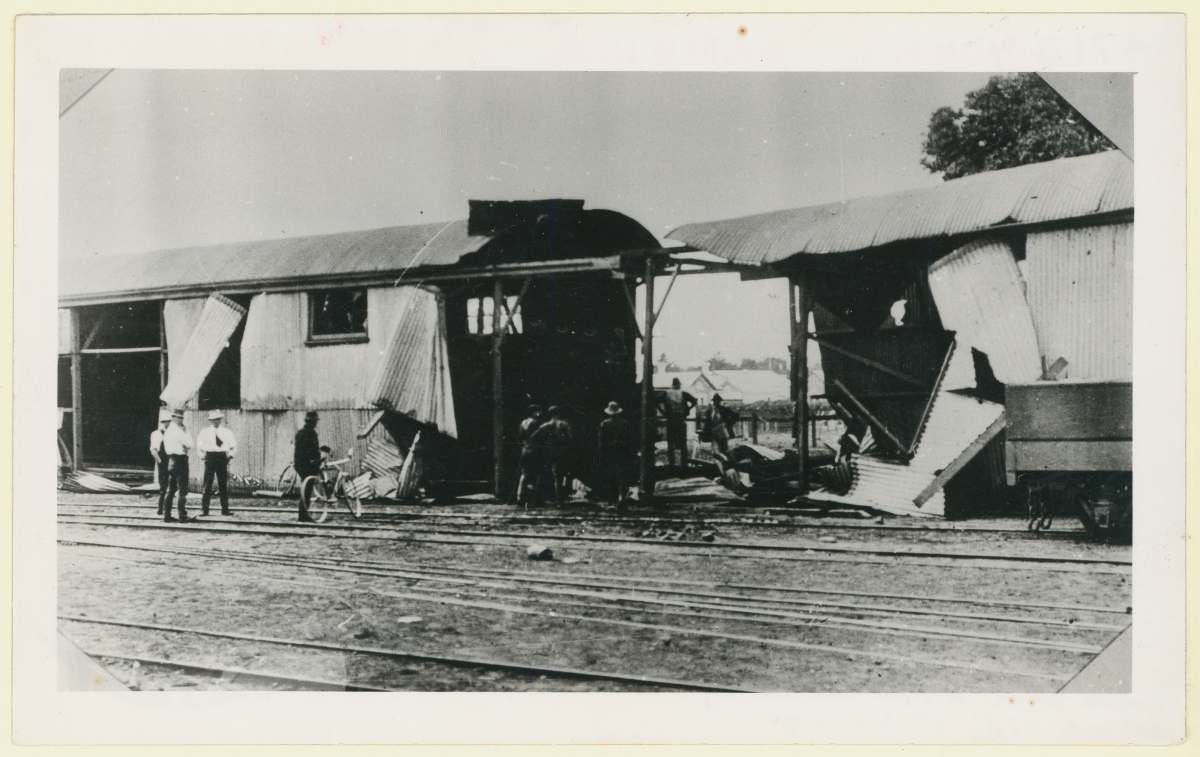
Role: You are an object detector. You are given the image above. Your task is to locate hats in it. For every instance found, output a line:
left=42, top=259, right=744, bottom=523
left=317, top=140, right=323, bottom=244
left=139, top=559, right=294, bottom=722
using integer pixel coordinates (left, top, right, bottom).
left=208, top=409, right=223, bottom=421
left=304, top=411, right=320, bottom=421
left=159, top=415, right=171, bottom=422
left=712, top=393, right=723, bottom=400
left=604, top=401, right=623, bottom=415
left=170, top=408, right=184, bottom=419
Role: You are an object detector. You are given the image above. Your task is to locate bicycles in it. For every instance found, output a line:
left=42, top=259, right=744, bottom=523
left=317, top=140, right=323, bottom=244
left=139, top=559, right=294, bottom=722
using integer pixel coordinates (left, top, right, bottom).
left=276, top=446, right=363, bottom=522
left=1028, top=500, right=1052, bottom=532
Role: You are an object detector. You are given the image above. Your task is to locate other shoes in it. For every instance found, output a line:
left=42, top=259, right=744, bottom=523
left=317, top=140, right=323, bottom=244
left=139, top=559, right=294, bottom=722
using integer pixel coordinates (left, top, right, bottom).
left=157, top=510, right=163, bottom=514
left=223, top=512, right=234, bottom=516
left=199, top=512, right=208, bottom=516
left=166, top=517, right=179, bottom=523
left=181, top=516, right=195, bottom=523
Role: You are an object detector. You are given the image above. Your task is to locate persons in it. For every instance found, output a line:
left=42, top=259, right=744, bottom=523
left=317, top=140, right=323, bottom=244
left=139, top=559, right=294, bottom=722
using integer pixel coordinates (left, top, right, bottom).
left=149, top=407, right=236, bottom=523
left=294, top=410, right=330, bottom=522
left=598, top=400, right=636, bottom=516
left=518, top=404, right=575, bottom=509
left=657, top=378, right=738, bottom=473
